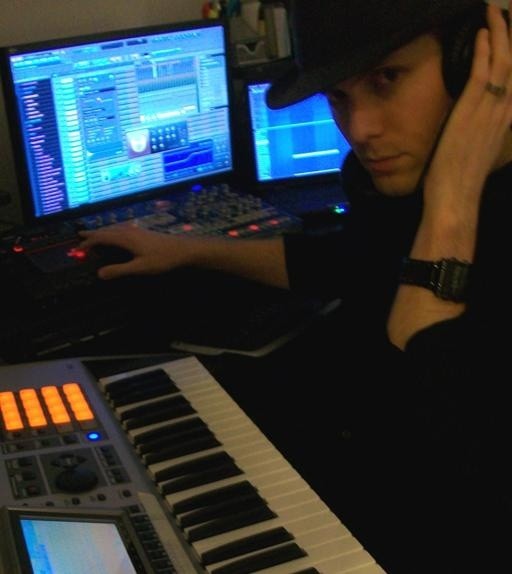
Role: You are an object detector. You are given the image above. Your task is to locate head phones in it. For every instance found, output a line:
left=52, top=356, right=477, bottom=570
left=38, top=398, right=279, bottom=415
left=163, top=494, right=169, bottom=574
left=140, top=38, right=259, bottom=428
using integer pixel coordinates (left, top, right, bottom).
left=442, top=9, right=510, bottom=100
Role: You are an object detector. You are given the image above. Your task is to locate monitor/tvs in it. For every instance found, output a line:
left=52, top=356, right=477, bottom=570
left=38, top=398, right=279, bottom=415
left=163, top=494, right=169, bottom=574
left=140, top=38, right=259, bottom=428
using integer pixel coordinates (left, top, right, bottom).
left=0, top=19, right=236, bottom=227
left=245, top=77, right=352, bottom=194
left=2, top=507, right=155, bottom=574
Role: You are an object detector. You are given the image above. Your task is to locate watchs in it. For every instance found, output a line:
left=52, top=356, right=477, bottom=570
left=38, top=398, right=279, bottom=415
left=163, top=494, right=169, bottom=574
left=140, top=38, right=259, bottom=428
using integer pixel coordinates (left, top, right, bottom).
left=394, top=252, right=482, bottom=303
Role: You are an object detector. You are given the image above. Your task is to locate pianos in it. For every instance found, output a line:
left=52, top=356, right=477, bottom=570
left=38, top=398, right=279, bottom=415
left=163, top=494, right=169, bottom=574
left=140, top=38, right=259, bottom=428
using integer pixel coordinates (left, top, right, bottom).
left=0, top=356, right=384, bottom=574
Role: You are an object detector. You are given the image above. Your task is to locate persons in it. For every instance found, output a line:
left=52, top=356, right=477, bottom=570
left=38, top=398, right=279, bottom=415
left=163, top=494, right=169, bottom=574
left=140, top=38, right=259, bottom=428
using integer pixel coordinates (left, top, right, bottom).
left=70, top=0, right=512, bottom=574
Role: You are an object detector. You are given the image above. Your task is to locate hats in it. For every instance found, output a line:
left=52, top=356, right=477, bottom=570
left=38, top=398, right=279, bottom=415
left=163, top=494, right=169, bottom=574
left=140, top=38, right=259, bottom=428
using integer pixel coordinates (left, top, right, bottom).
left=266, top=0, right=478, bottom=109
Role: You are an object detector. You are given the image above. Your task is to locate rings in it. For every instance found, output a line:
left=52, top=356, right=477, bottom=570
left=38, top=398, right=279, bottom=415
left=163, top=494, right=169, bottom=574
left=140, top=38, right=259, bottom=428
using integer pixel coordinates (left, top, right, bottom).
left=486, top=79, right=507, bottom=97
